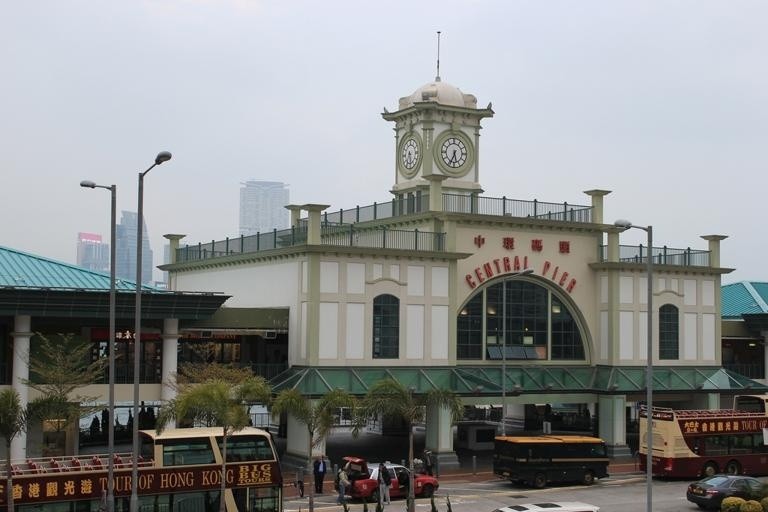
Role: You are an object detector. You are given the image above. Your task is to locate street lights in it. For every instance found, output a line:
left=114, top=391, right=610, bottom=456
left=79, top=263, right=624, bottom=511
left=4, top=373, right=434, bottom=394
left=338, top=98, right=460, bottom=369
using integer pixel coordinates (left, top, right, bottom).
left=615, top=218, right=656, bottom=512
left=80, top=179, right=116, bottom=510
left=129, top=152, right=172, bottom=511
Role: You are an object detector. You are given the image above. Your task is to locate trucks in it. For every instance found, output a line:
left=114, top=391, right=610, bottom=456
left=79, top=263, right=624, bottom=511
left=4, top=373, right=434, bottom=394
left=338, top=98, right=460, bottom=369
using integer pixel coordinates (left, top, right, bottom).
left=635, top=392, right=767, bottom=480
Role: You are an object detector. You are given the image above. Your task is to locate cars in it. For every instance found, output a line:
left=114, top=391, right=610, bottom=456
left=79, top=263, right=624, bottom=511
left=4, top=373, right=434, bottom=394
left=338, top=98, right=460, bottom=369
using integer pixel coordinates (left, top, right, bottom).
left=686, top=473, right=761, bottom=511
left=335, top=456, right=438, bottom=500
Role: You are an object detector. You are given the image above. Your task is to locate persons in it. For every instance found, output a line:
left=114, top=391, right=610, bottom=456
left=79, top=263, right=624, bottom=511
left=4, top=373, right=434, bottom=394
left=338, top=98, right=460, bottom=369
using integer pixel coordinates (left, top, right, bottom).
left=312, top=455, right=326, bottom=494
left=376, top=462, right=393, bottom=506
left=423, top=448, right=433, bottom=477
left=413, top=457, right=424, bottom=475
left=335, top=467, right=350, bottom=507
left=294, top=466, right=304, bottom=497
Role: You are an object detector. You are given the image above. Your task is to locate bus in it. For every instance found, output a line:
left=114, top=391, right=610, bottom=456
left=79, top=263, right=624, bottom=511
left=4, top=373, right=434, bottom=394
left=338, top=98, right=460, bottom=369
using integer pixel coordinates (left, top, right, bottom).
left=0, top=425, right=303, bottom=511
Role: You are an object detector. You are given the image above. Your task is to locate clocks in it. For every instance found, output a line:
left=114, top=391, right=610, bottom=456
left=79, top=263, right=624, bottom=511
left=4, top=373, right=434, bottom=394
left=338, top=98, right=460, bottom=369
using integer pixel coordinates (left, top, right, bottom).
left=433, top=126, right=476, bottom=179
left=397, top=131, right=423, bottom=178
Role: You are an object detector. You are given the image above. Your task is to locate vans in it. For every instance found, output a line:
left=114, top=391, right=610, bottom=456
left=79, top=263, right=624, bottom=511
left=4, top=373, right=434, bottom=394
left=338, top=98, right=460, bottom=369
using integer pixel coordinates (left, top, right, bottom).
left=493, top=433, right=610, bottom=489
